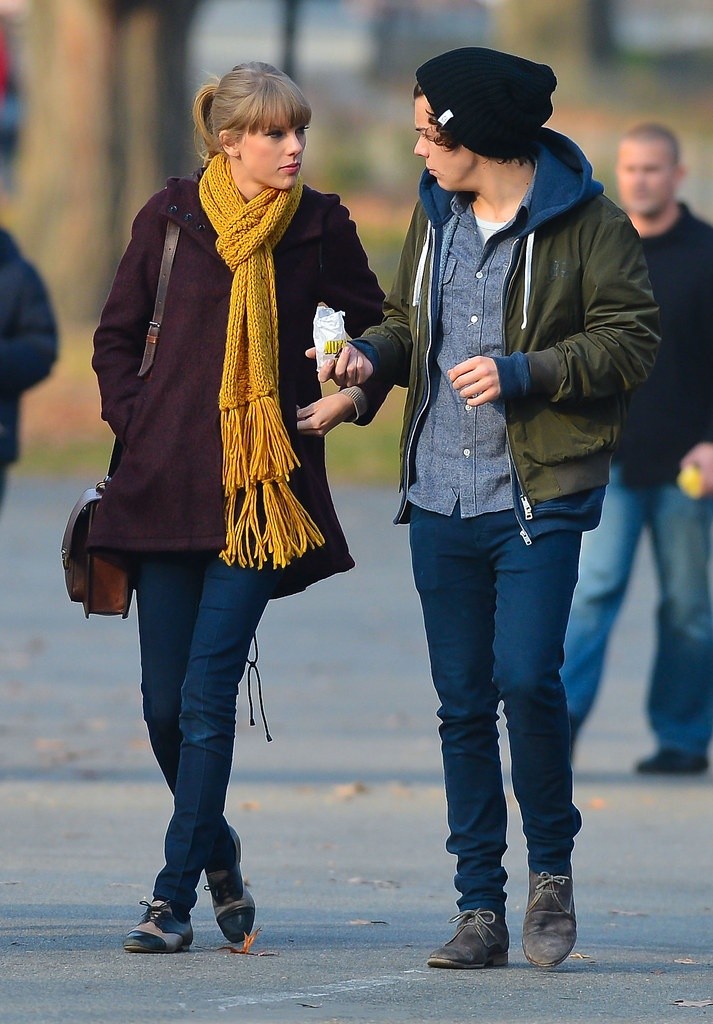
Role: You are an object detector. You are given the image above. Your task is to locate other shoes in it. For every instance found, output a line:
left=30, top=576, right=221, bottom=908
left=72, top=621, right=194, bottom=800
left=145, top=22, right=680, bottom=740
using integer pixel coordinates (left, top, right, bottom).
left=124, top=898, right=193, bottom=952
left=637, top=747, right=709, bottom=777
left=426, top=907, right=510, bottom=969
left=205, top=824, right=255, bottom=944
left=521, top=859, right=577, bottom=968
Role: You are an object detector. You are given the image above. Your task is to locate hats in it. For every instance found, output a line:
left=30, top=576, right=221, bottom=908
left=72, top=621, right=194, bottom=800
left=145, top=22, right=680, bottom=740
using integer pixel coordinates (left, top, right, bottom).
left=415, top=46, right=558, bottom=159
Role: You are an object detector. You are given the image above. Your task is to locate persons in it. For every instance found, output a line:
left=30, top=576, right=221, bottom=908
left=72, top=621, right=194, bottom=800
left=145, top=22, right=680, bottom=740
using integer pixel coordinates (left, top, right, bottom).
left=557, top=120, right=713, bottom=778
left=0, top=229, right=61, bottom=519
left=305, top=49, right=661, bottom=969
left=90, top=61, right=392, bottom=952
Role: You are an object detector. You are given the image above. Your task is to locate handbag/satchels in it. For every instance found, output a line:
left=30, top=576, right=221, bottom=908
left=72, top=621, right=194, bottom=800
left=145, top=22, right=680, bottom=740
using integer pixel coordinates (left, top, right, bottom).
left=61, top=476, right=133, bottom=621
